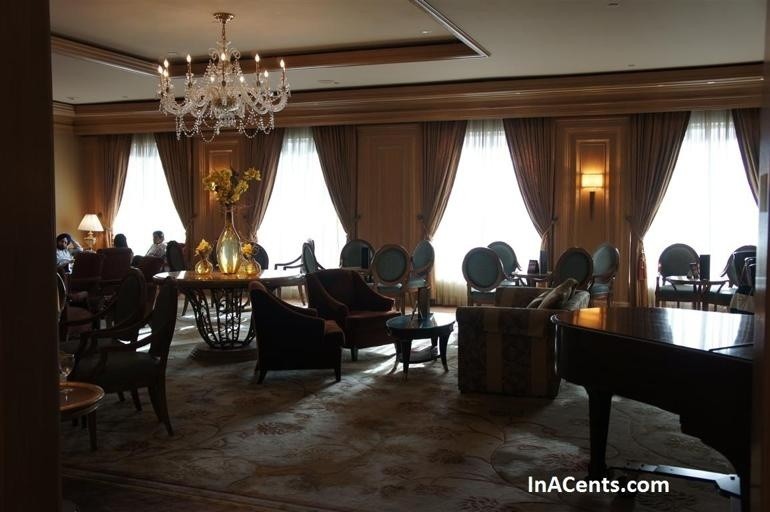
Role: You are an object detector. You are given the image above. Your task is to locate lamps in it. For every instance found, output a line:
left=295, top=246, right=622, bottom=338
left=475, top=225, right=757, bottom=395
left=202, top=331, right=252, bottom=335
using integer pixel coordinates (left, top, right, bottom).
left=580, top=165, right=605, bottom=223
left=76, top=214, right=103, bottom=253
left=153, top=12, right=291, bottom=141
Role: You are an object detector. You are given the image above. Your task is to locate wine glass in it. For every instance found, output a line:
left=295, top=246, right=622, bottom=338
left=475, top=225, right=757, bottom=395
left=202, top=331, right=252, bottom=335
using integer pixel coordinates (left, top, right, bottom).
left=62, top=387, right=75, bottom=403
left=59, top=353, right=76, bottom=394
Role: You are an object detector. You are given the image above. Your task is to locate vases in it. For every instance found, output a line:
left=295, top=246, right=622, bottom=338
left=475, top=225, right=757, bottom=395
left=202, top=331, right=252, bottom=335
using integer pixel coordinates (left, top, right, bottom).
left=216, top=206, right=242, bottom=274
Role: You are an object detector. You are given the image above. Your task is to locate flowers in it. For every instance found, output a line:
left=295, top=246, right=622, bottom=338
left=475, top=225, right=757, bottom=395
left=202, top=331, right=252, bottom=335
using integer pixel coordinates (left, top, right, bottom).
left=201, top=168, right=261, bottom=207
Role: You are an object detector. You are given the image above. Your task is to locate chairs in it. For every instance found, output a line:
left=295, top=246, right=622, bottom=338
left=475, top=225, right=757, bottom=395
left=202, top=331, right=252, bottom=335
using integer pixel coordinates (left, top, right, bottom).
left=56, top=237, right=755, bottom=437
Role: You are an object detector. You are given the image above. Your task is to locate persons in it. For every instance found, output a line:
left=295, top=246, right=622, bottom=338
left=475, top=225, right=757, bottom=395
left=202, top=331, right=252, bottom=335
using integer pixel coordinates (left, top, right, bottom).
left=56, top=233, right=83, bottom=274
left=113, top=233, right=128, bottom=248
left=130, top=230, right=167, bottom=266
